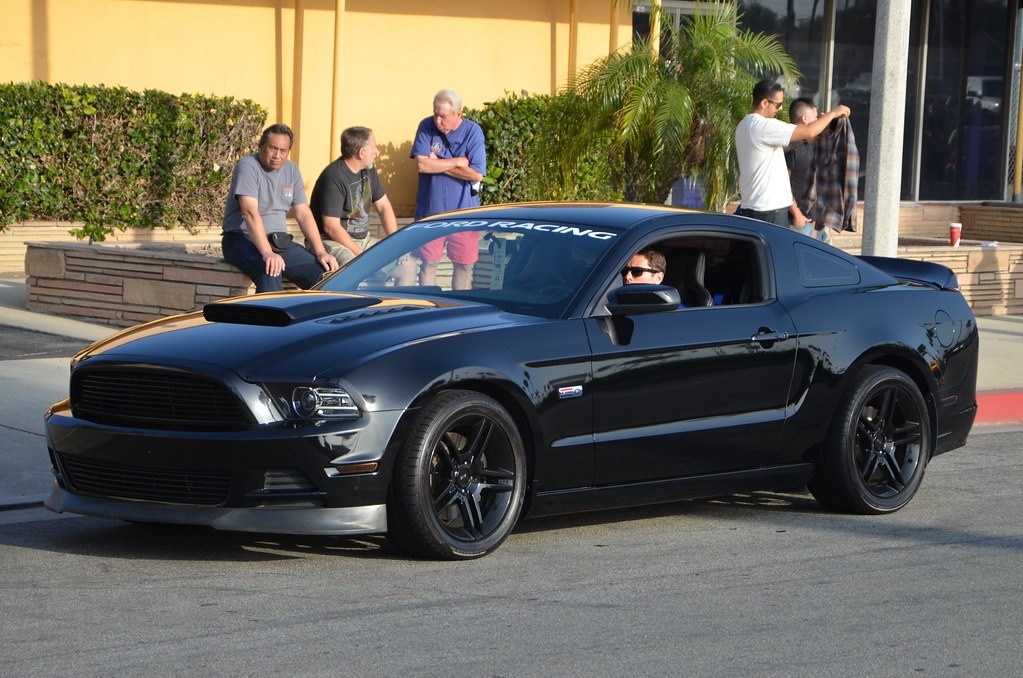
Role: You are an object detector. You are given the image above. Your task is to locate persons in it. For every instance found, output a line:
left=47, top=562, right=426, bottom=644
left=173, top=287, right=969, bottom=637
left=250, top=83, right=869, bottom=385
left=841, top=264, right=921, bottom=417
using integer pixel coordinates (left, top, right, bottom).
left=620, top=244, right=690, bottom=312
left=409, top=88, right=487, bottom=291
left=220, top=122, right=340, bottom=294
left=781, top=96, right=833, bottom=246
left=302, top=126, right=416, bottom=290
left=734, top=79, right=851, bottom=230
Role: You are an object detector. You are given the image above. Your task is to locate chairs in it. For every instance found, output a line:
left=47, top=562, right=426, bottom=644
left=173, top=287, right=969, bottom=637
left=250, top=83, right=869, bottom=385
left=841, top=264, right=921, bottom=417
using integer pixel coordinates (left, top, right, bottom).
left=663, top=245, right=713, bottom=308
left=504, top=241, right=581, bottom=290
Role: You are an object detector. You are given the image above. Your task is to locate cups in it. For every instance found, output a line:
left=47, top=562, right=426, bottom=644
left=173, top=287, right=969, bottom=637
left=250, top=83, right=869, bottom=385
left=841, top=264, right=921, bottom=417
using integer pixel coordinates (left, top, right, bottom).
left=949, top=223, right=962, bottom=247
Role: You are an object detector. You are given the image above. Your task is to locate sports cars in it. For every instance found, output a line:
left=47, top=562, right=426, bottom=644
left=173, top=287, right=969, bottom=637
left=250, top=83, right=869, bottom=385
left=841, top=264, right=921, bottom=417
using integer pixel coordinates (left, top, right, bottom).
left=44, top=201, right=980, bottom=561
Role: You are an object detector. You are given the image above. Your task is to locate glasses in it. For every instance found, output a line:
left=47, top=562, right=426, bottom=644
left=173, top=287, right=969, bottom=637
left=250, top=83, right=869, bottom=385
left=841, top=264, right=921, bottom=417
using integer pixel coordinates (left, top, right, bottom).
left=621, top=266, right=658, bottom=277
left=761, top=99, right=783, bottom=109
